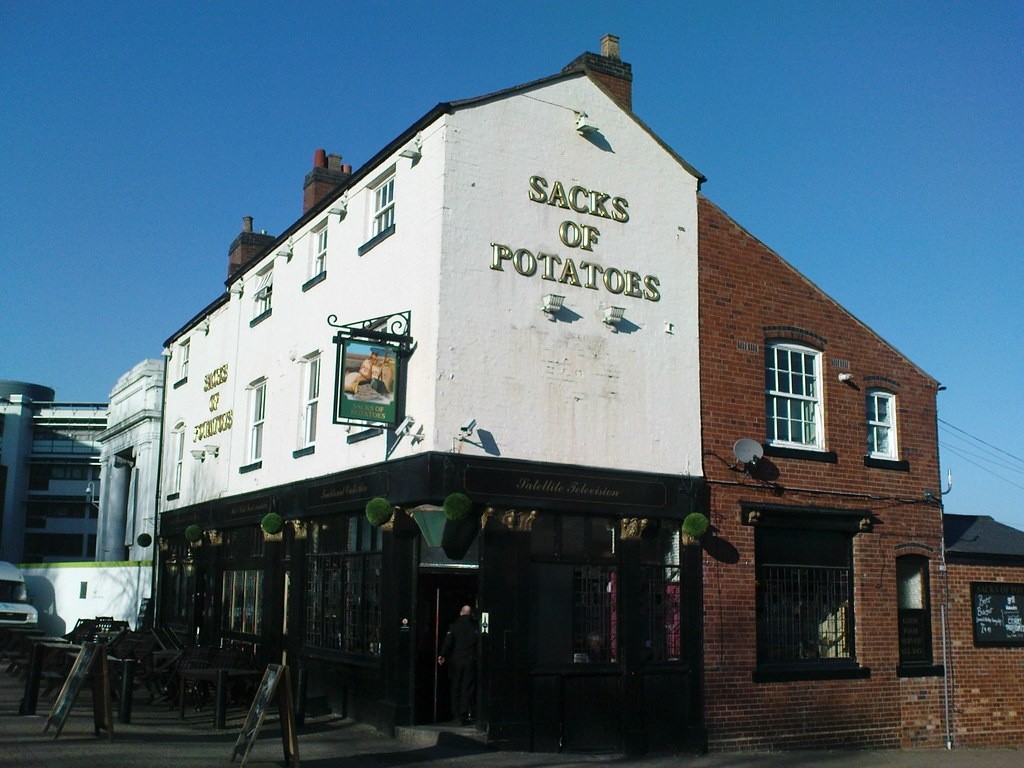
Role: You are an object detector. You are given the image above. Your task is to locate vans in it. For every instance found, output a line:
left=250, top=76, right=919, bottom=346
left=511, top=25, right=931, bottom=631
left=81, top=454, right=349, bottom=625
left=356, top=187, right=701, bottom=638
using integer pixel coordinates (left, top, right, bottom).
left=0, top=561, right=39, bottom=643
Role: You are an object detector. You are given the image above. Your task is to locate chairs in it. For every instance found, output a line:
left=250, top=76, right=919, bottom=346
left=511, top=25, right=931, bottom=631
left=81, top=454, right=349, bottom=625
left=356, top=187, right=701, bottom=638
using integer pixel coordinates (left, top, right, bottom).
left=6, top=615, right=256, bottom=705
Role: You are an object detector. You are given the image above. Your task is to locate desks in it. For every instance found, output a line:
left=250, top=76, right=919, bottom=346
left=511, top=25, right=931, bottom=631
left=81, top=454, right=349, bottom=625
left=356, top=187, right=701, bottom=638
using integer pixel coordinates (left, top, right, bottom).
left=67, top=651, right=136, bottom=724
left=7, top=627, right=44, bottom=634
left=26, top=635, right=67, bottom=644
left=24, top=642, right=85, bottom=716
left=175, top=667, right=265, bottom=731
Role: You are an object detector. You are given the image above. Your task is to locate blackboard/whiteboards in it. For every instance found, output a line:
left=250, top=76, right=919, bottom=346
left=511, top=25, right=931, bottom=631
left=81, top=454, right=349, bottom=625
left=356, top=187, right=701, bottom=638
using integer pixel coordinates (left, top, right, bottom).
left=234, top=663, right=299, bottom=759
left=46, top=641, right=113, bottom=730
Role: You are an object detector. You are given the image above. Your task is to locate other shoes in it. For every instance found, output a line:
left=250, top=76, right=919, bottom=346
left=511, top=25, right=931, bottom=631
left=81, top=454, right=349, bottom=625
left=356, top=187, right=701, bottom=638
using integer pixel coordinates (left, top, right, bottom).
left=465, top=712, right=472, bottom=722
left=447, top=719, right=463, bottom=726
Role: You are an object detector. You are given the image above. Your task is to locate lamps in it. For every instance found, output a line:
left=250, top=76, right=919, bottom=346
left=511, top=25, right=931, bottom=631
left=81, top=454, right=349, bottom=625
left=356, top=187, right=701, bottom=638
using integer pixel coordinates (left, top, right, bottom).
left=160, top=347, right=172, bottom=358
left=541, top=294, right=565, bottom=313
left=327, top=197, right=349, bottom=215
left=166, top=546, right=180, bottom=577
left=183, top=546, right=197, bottom=576
left=86, top=481, right=99, bottom=511
left=194, top=315, right=210, bottom=332
left=229, top=283, right=245, bottom=293
left=574, top=110, right=600, bottom=134
left=399, top=138, right=421, bottom=159
left=601, top=306, right=627, bottom=325
left=276, top=236, right=292, bottom=257
left=191, top=449, right=205, bottom=461
left=203, top=444, right=219, bottom=456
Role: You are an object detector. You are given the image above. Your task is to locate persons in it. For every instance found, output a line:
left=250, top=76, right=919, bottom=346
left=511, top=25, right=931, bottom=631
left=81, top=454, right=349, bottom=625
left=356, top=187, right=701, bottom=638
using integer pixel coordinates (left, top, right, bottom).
left=438, top=605, right=479, bottom=725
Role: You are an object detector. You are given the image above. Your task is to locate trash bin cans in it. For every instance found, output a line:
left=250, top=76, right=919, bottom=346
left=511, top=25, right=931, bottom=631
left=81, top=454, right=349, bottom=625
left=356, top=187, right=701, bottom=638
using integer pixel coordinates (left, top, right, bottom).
left=628, top=657, right=705, bottom=752
